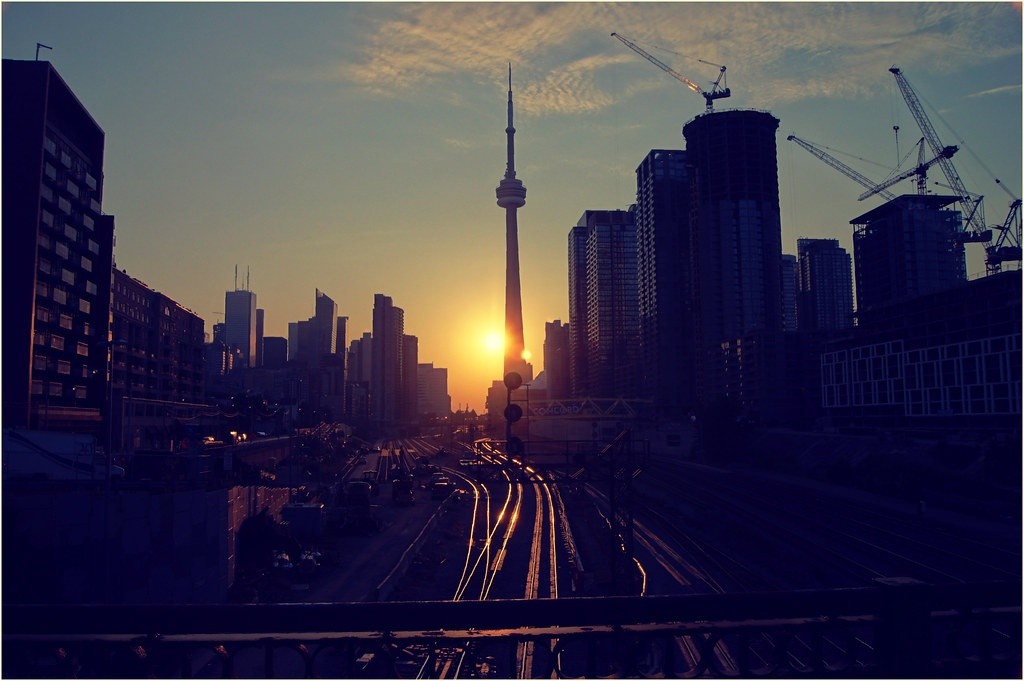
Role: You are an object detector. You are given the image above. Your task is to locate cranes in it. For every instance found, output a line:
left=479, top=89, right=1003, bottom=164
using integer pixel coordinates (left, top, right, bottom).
left=787, top=61, right=1022, bottom=279
left=611, top=31, right=730, bottom=112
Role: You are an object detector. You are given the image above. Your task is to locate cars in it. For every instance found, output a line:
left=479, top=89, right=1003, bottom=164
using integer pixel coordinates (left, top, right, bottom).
left=241, top=423, right=496, bottom=589
